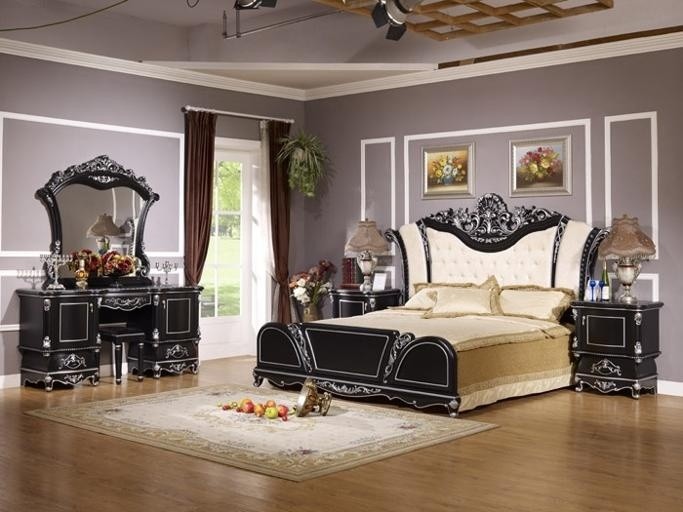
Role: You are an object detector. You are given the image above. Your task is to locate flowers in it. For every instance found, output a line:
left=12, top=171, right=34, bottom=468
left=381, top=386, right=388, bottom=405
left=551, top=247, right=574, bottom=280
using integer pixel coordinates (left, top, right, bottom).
left=70, top=250, right=100, bottom=275
left=520, top=146, right=561, bottom=181
left=430, top=154, right=466, bottom=184
left=89, top=250, right=142, bottom=277
left=288, top=259, right=337, bottom=307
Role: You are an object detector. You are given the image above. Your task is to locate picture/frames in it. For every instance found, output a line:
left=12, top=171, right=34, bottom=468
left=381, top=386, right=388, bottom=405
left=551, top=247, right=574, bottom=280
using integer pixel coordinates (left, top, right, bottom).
left=420, top=141, right=476, bottom=199
left=509, top=134, right=572, bottom=197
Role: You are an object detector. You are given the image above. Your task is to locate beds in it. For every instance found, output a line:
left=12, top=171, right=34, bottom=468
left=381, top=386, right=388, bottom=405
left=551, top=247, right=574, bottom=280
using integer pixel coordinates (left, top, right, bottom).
left=253, top=193, right=609, bottom=418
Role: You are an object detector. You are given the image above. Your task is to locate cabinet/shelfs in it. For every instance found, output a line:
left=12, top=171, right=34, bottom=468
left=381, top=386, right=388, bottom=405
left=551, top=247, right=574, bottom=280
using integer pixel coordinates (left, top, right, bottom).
left=15, top=286, right=204, bottom=392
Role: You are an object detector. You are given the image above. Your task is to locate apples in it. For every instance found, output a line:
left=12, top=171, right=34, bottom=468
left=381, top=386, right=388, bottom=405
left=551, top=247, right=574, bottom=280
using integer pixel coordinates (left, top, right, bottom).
left=222, top=399, right=288, bottom=419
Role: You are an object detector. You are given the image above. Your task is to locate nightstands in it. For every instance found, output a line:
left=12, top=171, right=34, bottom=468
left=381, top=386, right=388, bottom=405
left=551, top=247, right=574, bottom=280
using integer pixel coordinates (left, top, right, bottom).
left=568, top=301, right=664, bottom=399
left=329, top=289, right=400, bottom=318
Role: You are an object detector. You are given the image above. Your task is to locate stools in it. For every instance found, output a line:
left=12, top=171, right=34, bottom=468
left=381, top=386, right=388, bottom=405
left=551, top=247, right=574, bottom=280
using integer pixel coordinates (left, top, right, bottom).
left=96, top=327, right=145, bottom=384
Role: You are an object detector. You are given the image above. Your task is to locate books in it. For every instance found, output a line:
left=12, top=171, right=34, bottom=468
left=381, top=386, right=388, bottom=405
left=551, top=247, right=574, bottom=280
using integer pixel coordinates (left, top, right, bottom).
left=340, top=256, right=364, bottom=289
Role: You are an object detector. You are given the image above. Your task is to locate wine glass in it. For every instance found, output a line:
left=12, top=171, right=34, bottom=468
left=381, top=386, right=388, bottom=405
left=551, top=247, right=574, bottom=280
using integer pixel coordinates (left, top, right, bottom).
left=589, top=280, right=597, bottom=302
left=598, top=280, right=604, bottom=302
left=294, top=379, right=333, bottom=419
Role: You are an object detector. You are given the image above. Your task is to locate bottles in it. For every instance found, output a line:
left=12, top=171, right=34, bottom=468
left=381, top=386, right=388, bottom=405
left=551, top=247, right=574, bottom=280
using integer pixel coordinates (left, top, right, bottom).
left=74, top=259, right=90, bottom=292
left=600, top=260, right=610, bottom=300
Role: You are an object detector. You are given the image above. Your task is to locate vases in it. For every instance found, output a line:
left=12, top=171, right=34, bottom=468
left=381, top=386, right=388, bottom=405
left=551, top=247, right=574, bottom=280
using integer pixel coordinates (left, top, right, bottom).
left=303, top=303, right=318, bottom=322
left=106, top=273, right=129, bottom=287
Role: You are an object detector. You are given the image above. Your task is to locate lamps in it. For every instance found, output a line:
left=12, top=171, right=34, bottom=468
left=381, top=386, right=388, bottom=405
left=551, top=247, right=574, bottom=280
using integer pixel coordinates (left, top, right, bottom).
left=234, top=0, right=421, bottom=41
left=344, top=218, right=388, bottom=292
left=86, top=213, right=119, bottom=255
left=598, top=214, right=656, bottom=304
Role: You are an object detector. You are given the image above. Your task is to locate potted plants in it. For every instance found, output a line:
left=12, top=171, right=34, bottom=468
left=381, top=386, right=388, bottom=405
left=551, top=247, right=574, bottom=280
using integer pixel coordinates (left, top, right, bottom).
left=275, top=126, right=331, bottom=197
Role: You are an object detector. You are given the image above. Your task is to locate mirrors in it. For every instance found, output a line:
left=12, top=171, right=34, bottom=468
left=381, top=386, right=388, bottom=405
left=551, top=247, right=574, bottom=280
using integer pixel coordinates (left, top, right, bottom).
left=35, top=154, right=160, bottom=287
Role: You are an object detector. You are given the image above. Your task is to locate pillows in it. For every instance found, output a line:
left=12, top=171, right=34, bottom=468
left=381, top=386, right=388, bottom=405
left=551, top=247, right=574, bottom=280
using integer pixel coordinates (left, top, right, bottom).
left=404, top=275, right=576, bottom=322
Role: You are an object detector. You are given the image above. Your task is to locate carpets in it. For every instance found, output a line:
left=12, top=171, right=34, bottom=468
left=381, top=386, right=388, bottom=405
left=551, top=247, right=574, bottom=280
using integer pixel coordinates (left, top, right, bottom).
left=24, top=383, right=501, bottom=482
